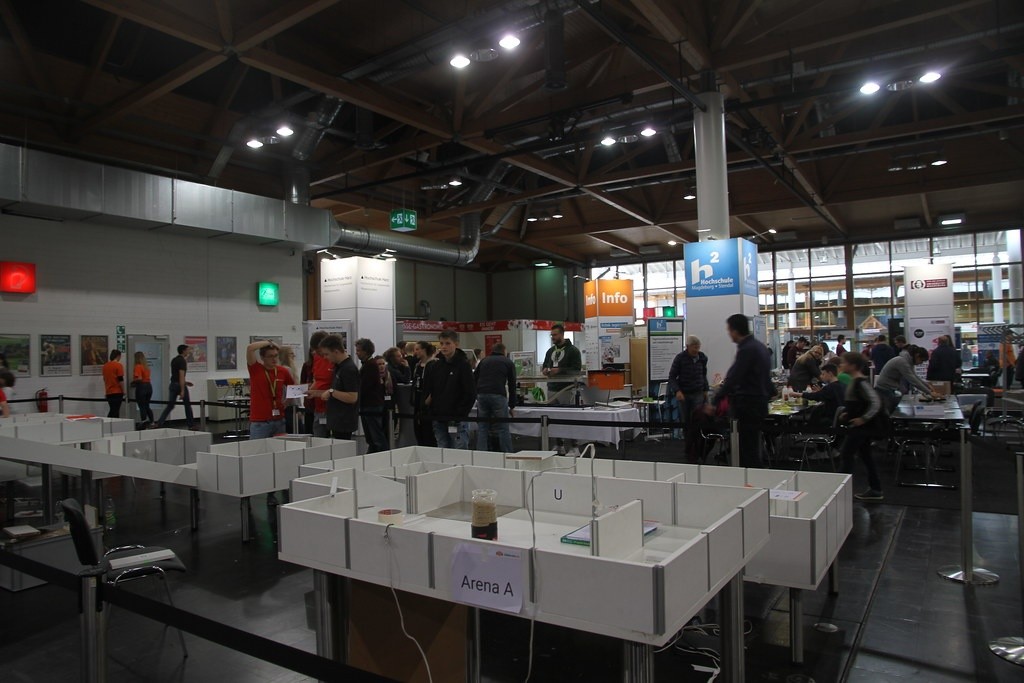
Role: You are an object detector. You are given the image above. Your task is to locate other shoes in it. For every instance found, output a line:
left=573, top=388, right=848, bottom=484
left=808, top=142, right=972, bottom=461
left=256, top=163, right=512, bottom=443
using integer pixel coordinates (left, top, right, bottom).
left=188, top=424, right=199, bottom=430
left=137, top=420, right=160, bottom=429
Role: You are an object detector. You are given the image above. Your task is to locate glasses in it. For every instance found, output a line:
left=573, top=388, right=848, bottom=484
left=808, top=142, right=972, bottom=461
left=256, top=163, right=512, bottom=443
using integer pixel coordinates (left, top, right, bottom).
left=264, top=353, right=279, bottom=359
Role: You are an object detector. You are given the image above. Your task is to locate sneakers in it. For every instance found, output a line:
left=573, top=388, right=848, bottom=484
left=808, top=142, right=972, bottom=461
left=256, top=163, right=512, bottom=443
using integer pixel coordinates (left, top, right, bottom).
left=854, top=486, right=884, bottom=500
left=807, top=447, right=841, bottom=459
left=551, top=445, right=566, bottom=455
left=565, top=447, right=581, bottom=457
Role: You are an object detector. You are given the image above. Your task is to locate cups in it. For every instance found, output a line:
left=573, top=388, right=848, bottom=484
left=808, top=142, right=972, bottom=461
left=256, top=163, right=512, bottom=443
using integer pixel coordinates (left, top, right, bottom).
left=470, top=488, right=498, bottom=542
left=788, top=400, right=795, bottom=405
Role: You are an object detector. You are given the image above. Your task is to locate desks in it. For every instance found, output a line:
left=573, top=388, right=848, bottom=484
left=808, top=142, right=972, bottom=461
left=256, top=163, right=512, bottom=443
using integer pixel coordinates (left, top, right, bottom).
left=889, top=395, right=964, bottom=490
left=757, top=397, right=826, bottom=468
left=451, top=405, right=639, bottom=455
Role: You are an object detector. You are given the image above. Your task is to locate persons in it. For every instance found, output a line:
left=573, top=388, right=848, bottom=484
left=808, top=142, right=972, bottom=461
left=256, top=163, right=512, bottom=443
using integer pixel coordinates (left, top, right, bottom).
left=0, top=353, right=16, bottom=418
left=542, top=325, right=582, bottom=457
left=155, top=344, right=201, bottom=430
left=132, top=352, right=155, bottom=428
left=668, top=313, right=1024, bottom=500
left=102, top=350, right=124, bottom=418
left=246, top=329, right=517, bottom=454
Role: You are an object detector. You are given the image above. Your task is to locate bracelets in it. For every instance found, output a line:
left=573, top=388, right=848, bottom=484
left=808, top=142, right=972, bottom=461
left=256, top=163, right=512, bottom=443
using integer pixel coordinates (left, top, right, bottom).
left=267, top=338, right=273, bottom=348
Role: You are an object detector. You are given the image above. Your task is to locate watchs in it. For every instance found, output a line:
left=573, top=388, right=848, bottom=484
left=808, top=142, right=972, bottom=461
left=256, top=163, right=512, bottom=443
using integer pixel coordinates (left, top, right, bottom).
left=329, top=388, right=334, bottom=398
left=547, top=368, right=552, bottom=377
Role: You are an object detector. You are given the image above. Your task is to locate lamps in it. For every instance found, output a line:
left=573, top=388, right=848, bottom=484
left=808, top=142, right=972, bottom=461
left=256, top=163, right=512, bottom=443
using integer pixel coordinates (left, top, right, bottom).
left=683, top=189, right=697, bottom=200
left=939, top=214, right=966, bottom=226
left=450, top=175, right=462, bottom=185
left=600, top=124, right=655, bottom=146
left=887, top=151, right=951, bottom=172
left=528, top=206, right=563, bottom=223
left=860, top=61, right=941, bottom=94
left=668, top=241, right=686, bottom=246
left=748, top=227, right=776, bottom=240
left=244, top=121, right=300, bottom=149
left=449, top=32, right=523, bottom=69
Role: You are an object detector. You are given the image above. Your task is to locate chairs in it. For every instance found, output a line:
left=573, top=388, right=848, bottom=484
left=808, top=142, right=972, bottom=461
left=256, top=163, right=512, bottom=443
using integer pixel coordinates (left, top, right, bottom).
left=895, top=399, right=1024, bottom=489
left=783, top=405, right=849, bottom=473
left=633, top=383, right=667, bottom=428
left=691, top=395, right=732, bottom=467
left=62, top=497, right=189, bottom=682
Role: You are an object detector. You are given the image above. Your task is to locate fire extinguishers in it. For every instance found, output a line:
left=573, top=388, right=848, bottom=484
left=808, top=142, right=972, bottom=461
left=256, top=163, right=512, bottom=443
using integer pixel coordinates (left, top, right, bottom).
left=35, top=387, right=49, bottom=412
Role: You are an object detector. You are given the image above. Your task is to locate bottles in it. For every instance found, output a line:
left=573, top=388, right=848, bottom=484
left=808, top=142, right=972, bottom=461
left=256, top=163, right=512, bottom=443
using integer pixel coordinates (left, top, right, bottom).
left=104, top=495, right=116, bottom=530
left=575, top=384, right=584, bottom=406
left=53, top=496, right=65, bottom=530
left=782, top=384, right=812, bottom=405
